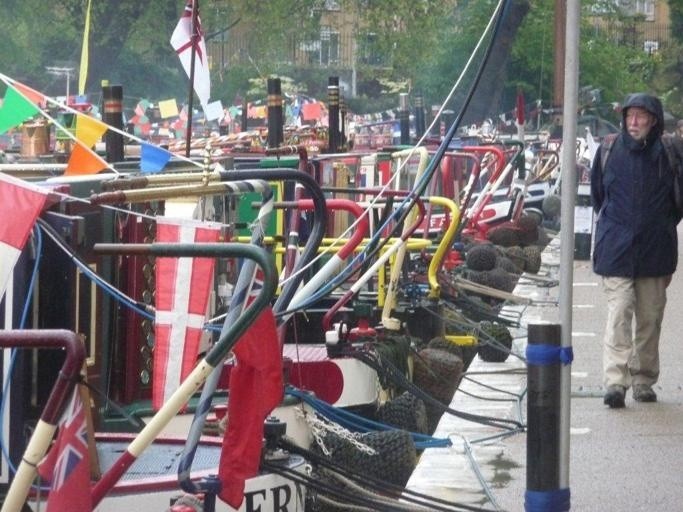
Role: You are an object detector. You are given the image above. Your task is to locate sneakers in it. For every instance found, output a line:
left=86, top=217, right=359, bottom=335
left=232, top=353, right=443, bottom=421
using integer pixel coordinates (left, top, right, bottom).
left=603, top=385, right=626, bottom=408
left=632, top=383, right=656, bottom=402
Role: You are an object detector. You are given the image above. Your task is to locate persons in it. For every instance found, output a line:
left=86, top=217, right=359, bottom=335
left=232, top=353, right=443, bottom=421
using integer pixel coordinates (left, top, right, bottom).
left=587, top=92, right=683, bottom=407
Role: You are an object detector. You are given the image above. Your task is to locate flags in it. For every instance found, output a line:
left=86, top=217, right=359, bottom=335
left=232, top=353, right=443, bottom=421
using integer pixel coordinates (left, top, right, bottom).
left=169, top=0, right=210, bottom=112
